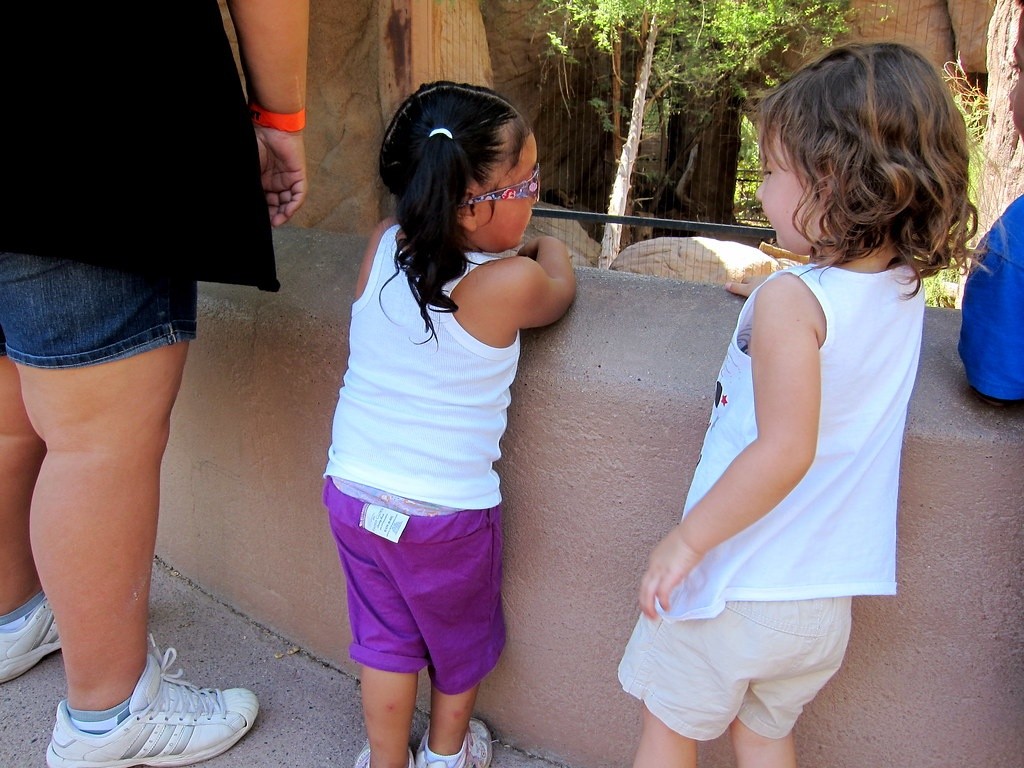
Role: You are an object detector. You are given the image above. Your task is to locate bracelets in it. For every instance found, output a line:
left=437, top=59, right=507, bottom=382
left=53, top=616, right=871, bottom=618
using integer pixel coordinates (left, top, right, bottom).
left=246, top=102, right=306, bottom=133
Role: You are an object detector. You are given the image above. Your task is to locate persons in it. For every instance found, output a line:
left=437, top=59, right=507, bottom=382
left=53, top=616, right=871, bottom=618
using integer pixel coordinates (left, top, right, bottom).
left=321, top=80, right=577, bottom=768
left=616, top=41, right=991, bottom=768
left=0, top=2, right=308, bottom=768
left=957, top=0, right=1024, bottom=401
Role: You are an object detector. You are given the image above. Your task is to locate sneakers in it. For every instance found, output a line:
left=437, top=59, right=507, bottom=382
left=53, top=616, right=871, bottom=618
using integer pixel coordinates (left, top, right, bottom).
left=0, top=596, right=61, bottom=684
left=46, top=635, right=259, bottom=768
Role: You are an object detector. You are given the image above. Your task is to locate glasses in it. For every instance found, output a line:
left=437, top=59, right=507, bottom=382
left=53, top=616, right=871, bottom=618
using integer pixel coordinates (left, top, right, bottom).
left=457, top=163, right=539, bottom=208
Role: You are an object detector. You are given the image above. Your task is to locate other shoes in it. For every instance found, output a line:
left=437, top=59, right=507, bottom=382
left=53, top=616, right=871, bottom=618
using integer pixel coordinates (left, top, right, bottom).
left=354, top=739, right=415, bottom=768
left=417, top=717, right=493, bottom=768
